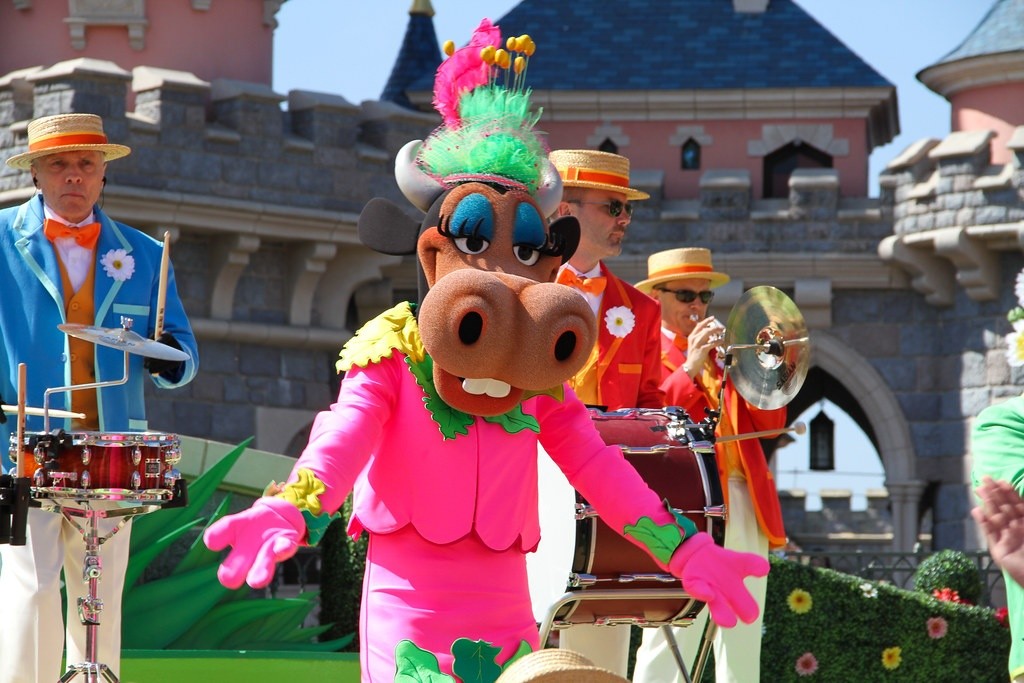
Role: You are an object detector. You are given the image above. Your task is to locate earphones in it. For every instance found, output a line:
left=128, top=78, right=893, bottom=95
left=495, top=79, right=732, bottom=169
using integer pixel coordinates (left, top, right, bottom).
left=33, top=177, right=38, bottom=187
left=102, top=176, right=106, bottom=186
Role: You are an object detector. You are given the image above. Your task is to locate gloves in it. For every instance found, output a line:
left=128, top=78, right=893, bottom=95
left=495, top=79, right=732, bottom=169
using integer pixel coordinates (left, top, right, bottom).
left=142, top=330, right=183, bottom=383
left=667, top=531, right=770, bottom=627
left=202, top=495, right=304, bottom=590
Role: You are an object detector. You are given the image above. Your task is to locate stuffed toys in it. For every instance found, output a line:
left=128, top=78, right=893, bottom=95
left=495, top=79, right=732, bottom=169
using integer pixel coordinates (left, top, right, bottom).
left=192, top=93, right=774, bottom=683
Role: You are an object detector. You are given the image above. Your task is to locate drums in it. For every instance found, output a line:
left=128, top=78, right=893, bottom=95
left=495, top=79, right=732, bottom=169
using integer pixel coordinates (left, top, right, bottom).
left=527, top=405, right=728, bottom=629
left=8, top=430, right=182, bottom=502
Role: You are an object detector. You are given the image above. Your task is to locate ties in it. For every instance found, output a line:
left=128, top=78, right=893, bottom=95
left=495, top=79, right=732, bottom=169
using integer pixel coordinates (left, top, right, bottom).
left=556, top=267, right=607, bottom=296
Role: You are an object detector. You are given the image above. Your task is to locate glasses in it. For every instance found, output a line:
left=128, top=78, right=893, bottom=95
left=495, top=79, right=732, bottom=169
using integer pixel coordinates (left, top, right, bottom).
left=657, top=285, right=714, bottom=304
left=567, top=199, right=633, bottom=216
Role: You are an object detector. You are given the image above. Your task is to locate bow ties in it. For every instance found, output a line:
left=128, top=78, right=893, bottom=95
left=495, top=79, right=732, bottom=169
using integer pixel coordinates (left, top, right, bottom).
left=44, top=218, right=101, bottom=250
left=673, top=333, right=688, bottom=351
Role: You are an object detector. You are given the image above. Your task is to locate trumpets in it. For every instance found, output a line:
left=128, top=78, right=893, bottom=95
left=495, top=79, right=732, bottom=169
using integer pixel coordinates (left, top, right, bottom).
left=689, top=314, right=724, bottom=365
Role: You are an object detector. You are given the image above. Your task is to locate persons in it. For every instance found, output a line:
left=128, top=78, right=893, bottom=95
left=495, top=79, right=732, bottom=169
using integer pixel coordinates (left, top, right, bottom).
left=0, top=111, right=205, bottom=683
left=526, top=141, right=670, bottom=683
left=960, top=386, right=1024, bottom=683
left=968, top=474, right=1024, bottom=588
left=626, top=240, right=792, bottom=682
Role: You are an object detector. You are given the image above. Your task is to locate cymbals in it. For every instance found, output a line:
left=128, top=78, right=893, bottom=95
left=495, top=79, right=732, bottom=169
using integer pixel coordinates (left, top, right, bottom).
left=57, top=322, right=192, bottom=362
left=724, top=285, right=812, bottom=411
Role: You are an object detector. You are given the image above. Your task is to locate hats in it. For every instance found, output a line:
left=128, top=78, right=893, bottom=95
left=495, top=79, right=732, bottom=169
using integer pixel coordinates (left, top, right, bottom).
left=4, top=112, right=130, bottom=169
left=493, top=648, right=630, bottom=683
left=632, top=246, right=729, bottom=296
left=547, top=148, right=650, bottom=200
